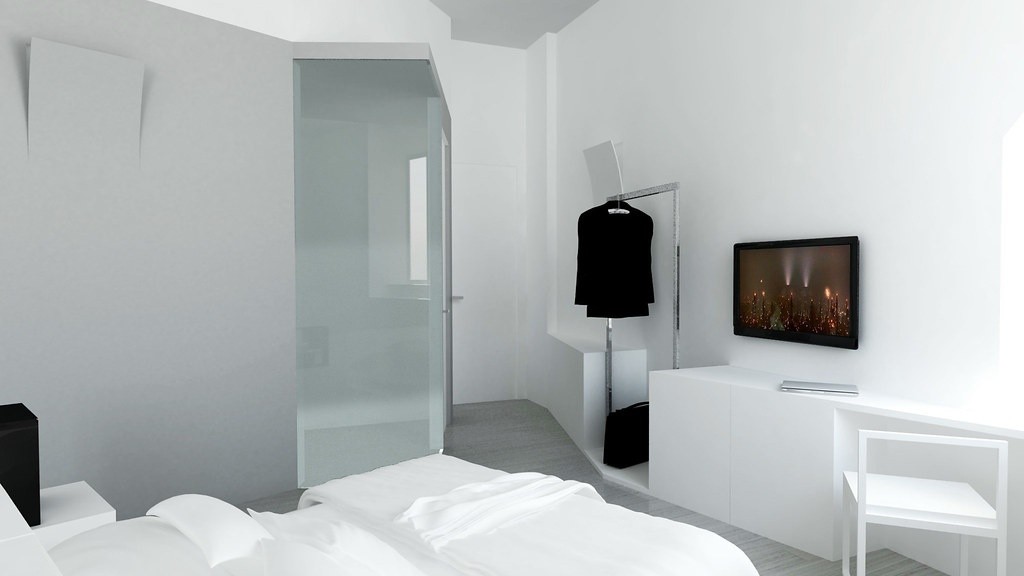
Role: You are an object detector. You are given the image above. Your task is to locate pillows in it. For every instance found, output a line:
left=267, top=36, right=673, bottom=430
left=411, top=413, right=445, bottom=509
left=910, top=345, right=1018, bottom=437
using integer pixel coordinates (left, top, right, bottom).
left=144, top=493, right=275, bottom=569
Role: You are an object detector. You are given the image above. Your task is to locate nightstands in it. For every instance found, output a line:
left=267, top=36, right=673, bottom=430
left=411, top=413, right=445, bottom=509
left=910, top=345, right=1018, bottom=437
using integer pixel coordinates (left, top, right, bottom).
left=31, top=480, right=118, bottom=554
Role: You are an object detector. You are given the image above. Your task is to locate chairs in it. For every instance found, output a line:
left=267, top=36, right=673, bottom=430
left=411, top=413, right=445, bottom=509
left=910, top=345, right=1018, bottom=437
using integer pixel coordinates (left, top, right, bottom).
left=839, top=430, right=1010, bottom=576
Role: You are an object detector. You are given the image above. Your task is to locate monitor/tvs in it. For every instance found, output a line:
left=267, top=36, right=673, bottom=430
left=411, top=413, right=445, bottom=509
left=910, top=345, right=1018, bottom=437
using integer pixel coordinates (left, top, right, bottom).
left=732, top=236, right=860, bottom=352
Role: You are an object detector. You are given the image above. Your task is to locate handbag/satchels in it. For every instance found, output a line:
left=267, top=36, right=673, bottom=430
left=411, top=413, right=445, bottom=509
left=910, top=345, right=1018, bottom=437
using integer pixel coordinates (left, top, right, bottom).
left=603, top=400, right=650, bottom=468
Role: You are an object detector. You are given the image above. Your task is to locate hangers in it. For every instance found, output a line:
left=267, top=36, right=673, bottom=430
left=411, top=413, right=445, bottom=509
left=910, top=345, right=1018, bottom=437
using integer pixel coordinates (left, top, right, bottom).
left=577, top=194, right=653, bottom=223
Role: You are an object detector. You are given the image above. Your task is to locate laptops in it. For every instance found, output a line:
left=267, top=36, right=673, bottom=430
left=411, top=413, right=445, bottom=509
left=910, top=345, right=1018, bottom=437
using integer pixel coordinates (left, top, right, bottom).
left=781, top=381, right=858, bottom=397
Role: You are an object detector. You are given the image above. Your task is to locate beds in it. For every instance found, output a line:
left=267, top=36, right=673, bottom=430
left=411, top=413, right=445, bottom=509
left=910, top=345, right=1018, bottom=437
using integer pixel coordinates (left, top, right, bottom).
left=47, top=450, right=761, bottom=576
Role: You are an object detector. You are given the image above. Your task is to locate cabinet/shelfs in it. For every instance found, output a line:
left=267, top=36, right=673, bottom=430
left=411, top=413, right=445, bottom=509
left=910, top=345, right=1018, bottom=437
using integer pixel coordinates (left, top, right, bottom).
left=648, top=370, right=836, bottom=562
